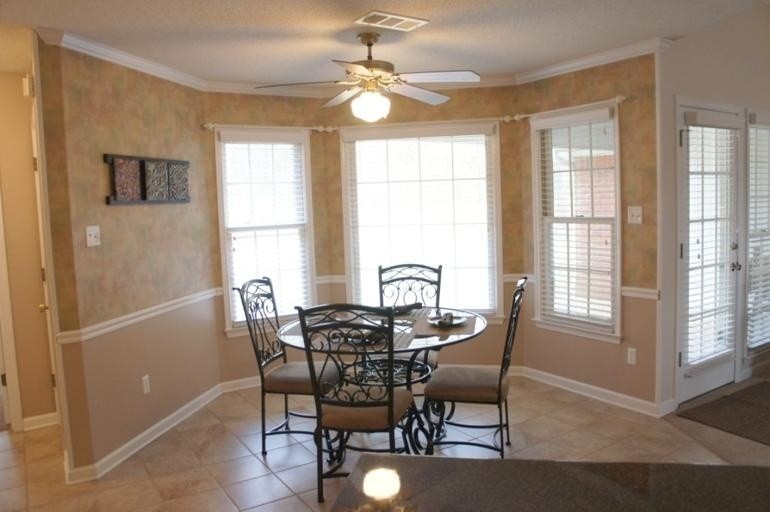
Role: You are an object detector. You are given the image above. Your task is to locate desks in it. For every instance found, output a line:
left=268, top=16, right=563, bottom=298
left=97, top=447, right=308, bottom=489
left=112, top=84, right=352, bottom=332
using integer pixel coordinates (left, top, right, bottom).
left=331, top=454, right=770, bottom=512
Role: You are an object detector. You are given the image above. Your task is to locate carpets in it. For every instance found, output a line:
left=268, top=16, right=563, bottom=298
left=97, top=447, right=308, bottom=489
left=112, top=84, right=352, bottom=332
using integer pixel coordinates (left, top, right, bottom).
left=677, top=380, right=770, bottom=447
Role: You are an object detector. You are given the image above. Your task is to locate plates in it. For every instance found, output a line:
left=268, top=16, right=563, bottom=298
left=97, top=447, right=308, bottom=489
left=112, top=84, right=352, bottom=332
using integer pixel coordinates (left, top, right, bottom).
left=427, top=315, right=467, bottom=327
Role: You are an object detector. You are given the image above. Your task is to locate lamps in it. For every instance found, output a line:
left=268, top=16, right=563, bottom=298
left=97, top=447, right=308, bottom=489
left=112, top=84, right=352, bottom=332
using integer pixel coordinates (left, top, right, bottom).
left=362, top=466, right=402, bottom=509
left=350, top=81, right=392, bottom=123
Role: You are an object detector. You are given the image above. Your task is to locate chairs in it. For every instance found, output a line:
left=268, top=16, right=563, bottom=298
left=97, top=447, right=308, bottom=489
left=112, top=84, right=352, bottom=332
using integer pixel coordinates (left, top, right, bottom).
left=423, top=276, right=529, bottom=458
left=232, top=275, right=345, bottom=463
left=364, top=263, right=444, bottom=437
left=294, top=303, right=412, bottom=502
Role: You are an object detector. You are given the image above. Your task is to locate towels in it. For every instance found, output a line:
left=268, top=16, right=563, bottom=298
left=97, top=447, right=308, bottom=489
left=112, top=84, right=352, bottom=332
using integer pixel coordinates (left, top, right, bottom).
left=438, top=312, right=453, bottom=328
left=332, top=329, right=385, bottom=342
left=384, top=302, right=423, bottom=316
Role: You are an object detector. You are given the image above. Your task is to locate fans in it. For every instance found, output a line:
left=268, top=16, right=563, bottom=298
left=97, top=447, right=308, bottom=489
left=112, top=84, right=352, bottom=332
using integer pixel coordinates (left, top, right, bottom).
left=254, top=33, right=481, bottom=104
